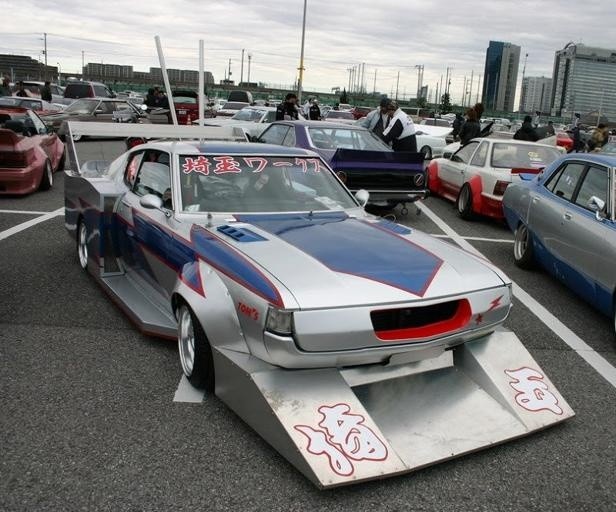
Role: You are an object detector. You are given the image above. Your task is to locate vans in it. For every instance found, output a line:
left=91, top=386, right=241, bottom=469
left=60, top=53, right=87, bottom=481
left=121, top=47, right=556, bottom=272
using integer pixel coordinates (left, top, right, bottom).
left=171, top=89, right=217, bottom=125
left=63, top=81, right=114, bottom=100
left=227, top=89, right=254, bottom=106
left=399, top=105, right=425, bottom=121
left=9, top=81, right=64, bottom=100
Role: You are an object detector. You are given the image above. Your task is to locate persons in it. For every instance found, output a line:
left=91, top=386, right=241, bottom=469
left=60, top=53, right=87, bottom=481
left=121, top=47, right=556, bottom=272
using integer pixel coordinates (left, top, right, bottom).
left=453, top=103, right=490, bottom=146
left=14, top=79, right=30, bottom=97
left=360, top=98, right=417, bottom=153
left=0, top=73, right=13, bottom=98
left=515, top=112, right=609, bottom=153
left=275, top=93, right=324, bottom=121
left=429, top=111, right=441, bottom=118
left=334, top=103, right=339, bottom=110
left=143, top=85, right=169, bottom=113
left=40, top=79, right=53, bottom=104
left=349, top=107, right=354, bottom=112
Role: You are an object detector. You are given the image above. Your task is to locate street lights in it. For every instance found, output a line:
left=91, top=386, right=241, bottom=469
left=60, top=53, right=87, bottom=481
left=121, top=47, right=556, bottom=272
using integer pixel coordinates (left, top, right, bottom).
left=247, top=51, right=253, bottom=82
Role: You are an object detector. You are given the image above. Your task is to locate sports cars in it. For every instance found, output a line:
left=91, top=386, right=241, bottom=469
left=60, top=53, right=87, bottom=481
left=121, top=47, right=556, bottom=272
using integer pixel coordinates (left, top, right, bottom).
left=0, top=106, right=65, bottom=197
left=59, top=120, right=576, bottom=490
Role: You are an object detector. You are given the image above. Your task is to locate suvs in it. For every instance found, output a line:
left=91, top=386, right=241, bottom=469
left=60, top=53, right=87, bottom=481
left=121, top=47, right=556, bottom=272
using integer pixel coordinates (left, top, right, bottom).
left=352, top=106, right=372, bottom=120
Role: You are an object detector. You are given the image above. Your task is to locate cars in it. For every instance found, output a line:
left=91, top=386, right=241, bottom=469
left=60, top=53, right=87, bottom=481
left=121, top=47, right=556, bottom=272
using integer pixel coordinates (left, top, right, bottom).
left=231, top=106, right=306, bottom=126
left=433, top=112, right=457, bottom=126
left=3, top=96, right=52, bottom=115
left=426, top=135, right=562, bottom=221
left=245, top=120, right=427, bottom=225
left=205, top=99, right=251, bottom=116
left=321, top=110, right=355, bottom=121
left=337, top=103, right=356, bottom=113
left=39, top=97, right=145, bottom=133
left=500, top=153, right=616, bottom=323
